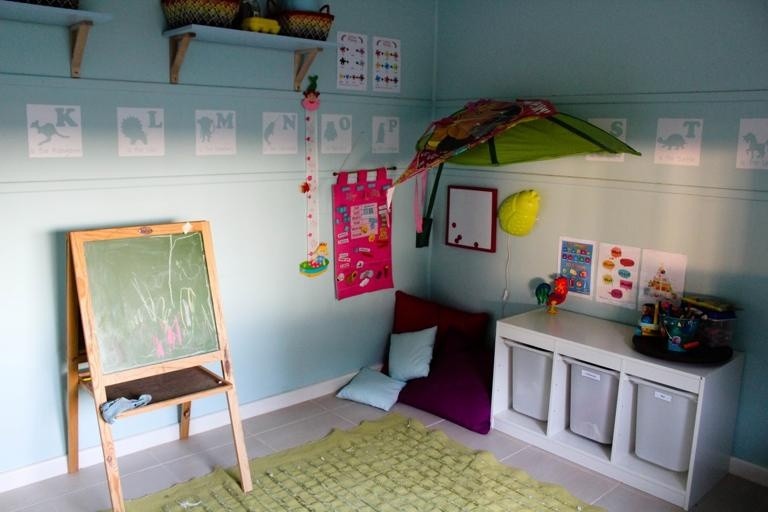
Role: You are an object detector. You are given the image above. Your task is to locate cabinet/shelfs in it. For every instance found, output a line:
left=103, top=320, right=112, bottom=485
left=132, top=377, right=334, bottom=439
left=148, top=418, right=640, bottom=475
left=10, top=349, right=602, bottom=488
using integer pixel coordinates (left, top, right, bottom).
left=490, top=305, right=743, bottom=511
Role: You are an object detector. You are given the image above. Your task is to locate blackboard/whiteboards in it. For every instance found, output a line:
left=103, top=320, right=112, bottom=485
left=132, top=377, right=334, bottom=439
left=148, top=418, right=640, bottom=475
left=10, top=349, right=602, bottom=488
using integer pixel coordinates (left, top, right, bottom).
left=70, top=220, right=228, bottom=388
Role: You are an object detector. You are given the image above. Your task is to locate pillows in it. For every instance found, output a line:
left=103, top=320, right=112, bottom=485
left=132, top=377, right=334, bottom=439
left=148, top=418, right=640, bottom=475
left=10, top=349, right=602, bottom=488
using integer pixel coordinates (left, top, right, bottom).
left=335, top=365, right=405, bottom=412
left=387, top=327, right=437, bottom=379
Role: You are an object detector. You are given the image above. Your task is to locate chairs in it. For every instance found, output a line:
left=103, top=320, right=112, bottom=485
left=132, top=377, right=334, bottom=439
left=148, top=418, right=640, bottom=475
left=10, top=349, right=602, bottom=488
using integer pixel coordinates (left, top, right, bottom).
left=384, top=288, right=497, bottom=434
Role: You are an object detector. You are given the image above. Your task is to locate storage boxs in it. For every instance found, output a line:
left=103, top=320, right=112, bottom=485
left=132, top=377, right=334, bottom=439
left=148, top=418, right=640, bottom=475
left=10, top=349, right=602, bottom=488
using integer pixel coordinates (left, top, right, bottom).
left=560, top=358, right=618, bottom=448
left=505, top=342, right=552, bottom=422
left=627, top=376, right=697, bottom=478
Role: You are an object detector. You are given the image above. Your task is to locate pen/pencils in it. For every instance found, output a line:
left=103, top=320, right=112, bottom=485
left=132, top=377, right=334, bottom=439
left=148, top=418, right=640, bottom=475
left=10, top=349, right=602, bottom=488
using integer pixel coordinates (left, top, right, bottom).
left=667, top=302, right=695, bottom=319
left=653, top=301, right=661, bottom=324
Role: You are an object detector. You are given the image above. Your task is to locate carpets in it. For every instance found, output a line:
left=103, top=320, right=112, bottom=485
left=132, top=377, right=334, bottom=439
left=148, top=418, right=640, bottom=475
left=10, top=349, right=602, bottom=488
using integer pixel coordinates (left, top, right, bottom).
left=111, top=412, right=596, bottom=510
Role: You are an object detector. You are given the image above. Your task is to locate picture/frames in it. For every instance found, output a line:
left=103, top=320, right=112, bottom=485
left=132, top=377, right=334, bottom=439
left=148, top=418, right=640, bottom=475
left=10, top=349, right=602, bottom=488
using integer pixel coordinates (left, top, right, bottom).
left=557, top=237, right=597, bottom=298
